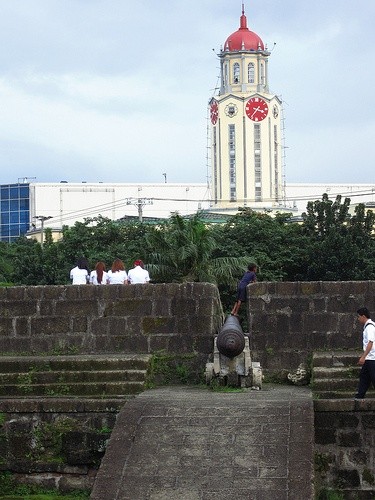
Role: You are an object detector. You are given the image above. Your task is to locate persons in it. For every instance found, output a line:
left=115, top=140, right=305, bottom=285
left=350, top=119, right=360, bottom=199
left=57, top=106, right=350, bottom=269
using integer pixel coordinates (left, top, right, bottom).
left=127, top=260, right=150, bottom=284
left=107, top=258, right=128, bottom=284
left=90, top=262, right=109, bottom=285
left=353, top=307, right=375, bottom=398
left=70, top=257, right=89, bottom=285
left=231, top=262, right=257, bottom=317
